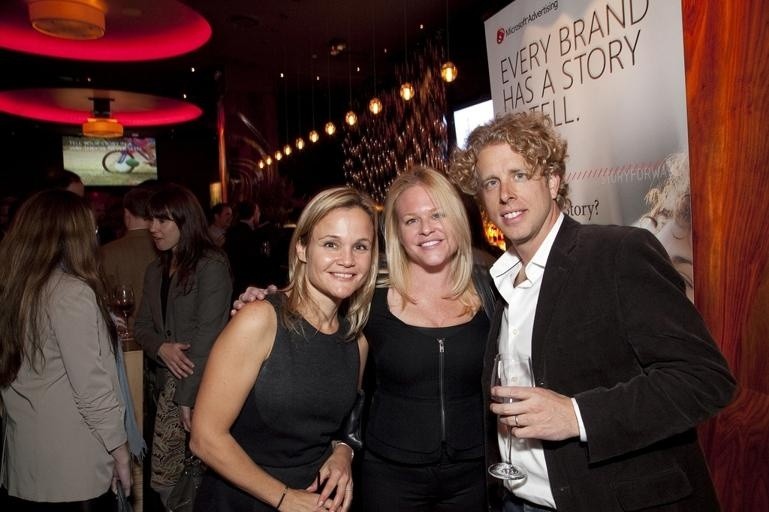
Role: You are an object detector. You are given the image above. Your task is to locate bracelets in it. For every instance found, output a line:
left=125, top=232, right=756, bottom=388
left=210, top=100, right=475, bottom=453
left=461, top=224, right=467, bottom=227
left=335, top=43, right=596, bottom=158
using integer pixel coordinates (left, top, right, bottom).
left=331, top=442, right=356, bottom=458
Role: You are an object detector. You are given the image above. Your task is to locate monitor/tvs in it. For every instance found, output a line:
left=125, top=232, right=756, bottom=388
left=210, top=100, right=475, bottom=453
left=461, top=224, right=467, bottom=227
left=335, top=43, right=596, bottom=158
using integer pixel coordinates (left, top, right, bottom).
left=62, top=135, right=158, bottom=187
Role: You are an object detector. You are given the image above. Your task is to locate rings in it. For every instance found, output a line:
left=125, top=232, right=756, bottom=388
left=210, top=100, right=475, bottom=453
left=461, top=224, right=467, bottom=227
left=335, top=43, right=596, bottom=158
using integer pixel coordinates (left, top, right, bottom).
left=514, top=416, right=519, bottom=425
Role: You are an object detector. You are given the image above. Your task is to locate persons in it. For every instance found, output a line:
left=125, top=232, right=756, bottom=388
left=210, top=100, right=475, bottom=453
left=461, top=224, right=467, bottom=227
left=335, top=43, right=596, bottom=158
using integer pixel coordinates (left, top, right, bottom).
left=136, top=184, right=233, bottom=512
left=11, top=169, right=84, bottom=198
left=446, top=113, right=738, bottom=512
left=616, top=155, right=693, bottom=304
left=229, top=163, right=508, bottom=511
left=91, top=187, right=167, bottom=451
left=207, top=204, right=232, bottom=248
left=188, top=187, right=380, bottom=512
left=1, top=191, right=133, bottom=511
left=232, top=202, right=276, bottom=296
